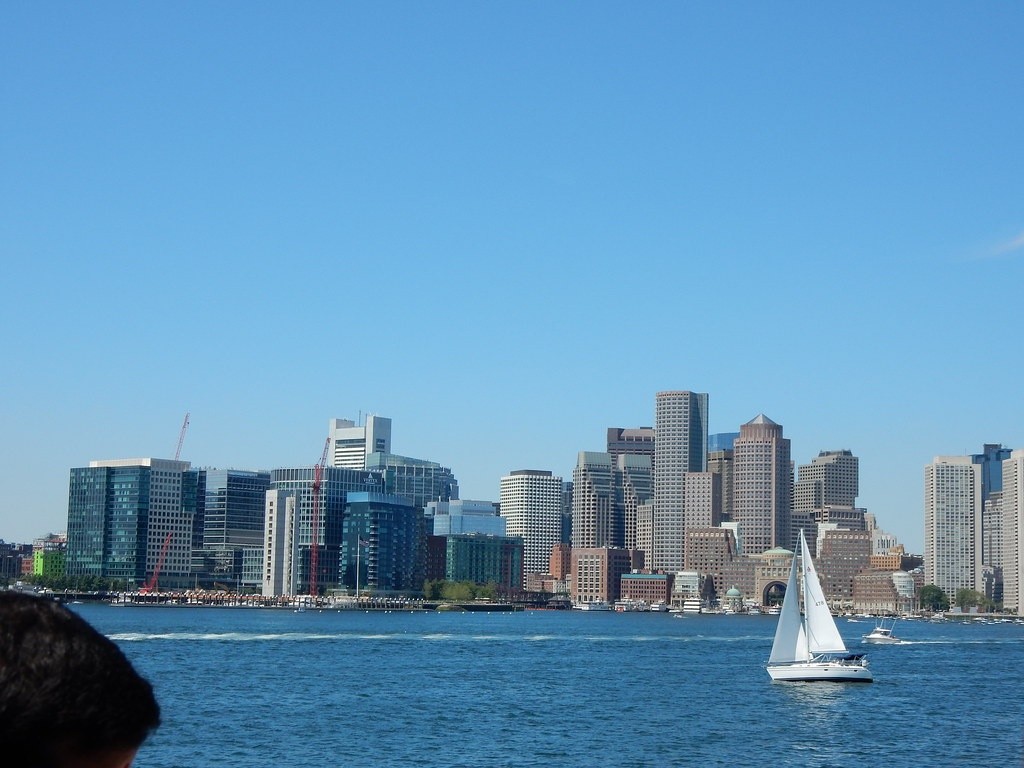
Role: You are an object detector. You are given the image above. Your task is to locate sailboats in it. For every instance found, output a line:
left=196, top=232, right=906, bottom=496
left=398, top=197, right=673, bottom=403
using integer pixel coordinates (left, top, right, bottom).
left=962, top=605, right=1024, bottom=625
left=766, top=529, right=873, bottom=683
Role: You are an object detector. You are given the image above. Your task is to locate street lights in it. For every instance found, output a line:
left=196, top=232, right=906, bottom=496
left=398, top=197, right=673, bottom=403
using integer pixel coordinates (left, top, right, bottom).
left=908, top=595, right=911, bottom=618
left=357, top=534, right=368, bottom=601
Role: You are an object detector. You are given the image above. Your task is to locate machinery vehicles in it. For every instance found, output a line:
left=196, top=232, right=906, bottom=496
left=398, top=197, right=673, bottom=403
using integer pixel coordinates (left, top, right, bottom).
left=213, top=582, right=238, bottom=594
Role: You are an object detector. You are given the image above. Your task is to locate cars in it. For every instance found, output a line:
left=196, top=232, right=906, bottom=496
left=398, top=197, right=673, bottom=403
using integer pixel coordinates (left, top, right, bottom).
left=723, top=605, right=730, bottom=610
left=38, top=590, right=53, bottom=594
left=842, top=602, right=852, bottom=608
left=317, top=601, right=322, bottom=606
left=255, top=600, right=261, bottom=604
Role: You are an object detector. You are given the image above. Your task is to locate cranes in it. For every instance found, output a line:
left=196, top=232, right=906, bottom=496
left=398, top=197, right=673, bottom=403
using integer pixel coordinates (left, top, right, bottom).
left=175, top=412, right=191, bottom=460
left=139, top=532, right=171, bottom=593
left=310, top=437, right=331, bottom=596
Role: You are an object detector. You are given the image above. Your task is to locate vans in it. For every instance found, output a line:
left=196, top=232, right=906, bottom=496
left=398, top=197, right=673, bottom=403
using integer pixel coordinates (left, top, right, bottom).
left=749, top=609, right=761, bottom=613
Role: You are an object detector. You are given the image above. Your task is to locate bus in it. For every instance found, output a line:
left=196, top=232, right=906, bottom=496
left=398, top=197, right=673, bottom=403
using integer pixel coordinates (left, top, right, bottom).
left=726, top=610, right=735, bottom=614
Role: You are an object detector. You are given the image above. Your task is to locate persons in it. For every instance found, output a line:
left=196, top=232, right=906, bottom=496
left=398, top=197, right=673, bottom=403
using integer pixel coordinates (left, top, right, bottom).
left=0, top=589, right=162, bottom=768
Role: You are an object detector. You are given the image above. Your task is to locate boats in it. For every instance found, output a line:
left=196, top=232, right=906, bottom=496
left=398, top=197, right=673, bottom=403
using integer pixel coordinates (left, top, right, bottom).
left=848, top=615, right=870, bottom=622
left=861, top=602, right=901, bottom=643
left=294, top=608, right=306, bottom=613
